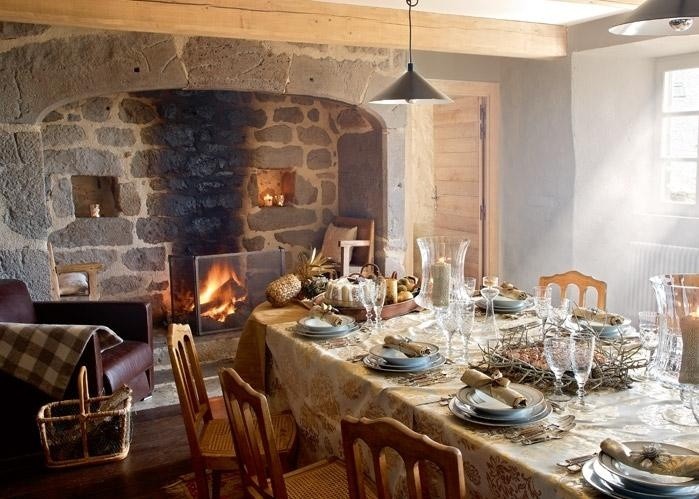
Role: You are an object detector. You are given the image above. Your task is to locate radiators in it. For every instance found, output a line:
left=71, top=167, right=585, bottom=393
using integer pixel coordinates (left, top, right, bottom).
left=621, top=238, right=698, bottom=323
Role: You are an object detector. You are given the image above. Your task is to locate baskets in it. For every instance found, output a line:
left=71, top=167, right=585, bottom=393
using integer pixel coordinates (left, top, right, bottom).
left=36, top=364, right=133, bottom=468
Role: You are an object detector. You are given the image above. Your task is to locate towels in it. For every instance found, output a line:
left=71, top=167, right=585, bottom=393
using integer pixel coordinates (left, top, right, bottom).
left=459, top=368, right=528, bottom=412
left=301, top=296, right=345, bottom=327
left=591, top=431, right=698, bottom=485
left=492, top=280, right=530, bottom=304
left=571, top=306, right=625, bottom=328
left=380, top=332, right=433, bottom=360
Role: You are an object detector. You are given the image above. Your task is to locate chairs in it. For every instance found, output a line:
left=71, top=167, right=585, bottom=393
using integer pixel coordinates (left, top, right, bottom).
left=216, top=364, right=352, bottom=497
left=340, top=412, right=468, bottom=497
left=295, top=214, right=379, bottom=288
left=665, top=272, right=698, bottom=337
left=169, top=318, right=301, bottom=499
left=534, top=270, right=608, bottom=315
left=2, top=274, right=159, bottom=418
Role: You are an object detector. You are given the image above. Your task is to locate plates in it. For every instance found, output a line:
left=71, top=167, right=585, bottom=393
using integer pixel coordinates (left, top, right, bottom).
left=286, top=291, right=535, bottom=375
left=566, top=313, right=634, bottom=339
left=443, top=378, right=699, bottom=499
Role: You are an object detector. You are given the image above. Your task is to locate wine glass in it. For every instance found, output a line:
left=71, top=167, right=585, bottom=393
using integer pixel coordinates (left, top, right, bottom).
left=548, top=296, right=572, bottom=336
left=358, top=234, right=500, bottom=366
left=638, top=271, right=699, bottom=426
left=531, top=286, right=550, bottom=338
left=570, top=330, right=596, bottom=413
left=543, top=336, right=568, bottom=403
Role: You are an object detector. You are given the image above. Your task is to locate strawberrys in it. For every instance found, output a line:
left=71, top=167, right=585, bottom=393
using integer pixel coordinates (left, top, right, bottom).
left=507, top=347, right=606, bottom=371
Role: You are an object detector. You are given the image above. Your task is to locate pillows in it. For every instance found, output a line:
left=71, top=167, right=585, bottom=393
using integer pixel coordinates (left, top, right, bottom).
left=318, top=220, right=362, bottom=264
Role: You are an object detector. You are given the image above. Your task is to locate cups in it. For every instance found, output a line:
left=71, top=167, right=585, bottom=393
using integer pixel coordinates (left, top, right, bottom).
left=89, top=203, right=101, bottom=218
left=275, top=194, right=285, bottom=206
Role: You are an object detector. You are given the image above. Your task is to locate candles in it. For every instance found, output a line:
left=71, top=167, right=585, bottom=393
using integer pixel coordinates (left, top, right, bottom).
left=264, top=194, right=274, bottom=207
left=430, top=260, right=451, bottom=311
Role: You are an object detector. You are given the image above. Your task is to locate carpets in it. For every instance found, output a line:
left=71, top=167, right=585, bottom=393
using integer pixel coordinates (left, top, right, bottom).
left=159, top=386, right=254, bottom=499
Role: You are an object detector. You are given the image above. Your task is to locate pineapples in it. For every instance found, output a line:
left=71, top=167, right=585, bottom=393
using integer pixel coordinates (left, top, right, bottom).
left=264, top=247, right=336, bottom=307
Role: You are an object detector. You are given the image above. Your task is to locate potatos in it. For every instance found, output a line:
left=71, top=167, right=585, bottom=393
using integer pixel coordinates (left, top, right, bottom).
left=398, top=278, right=413, bottom=301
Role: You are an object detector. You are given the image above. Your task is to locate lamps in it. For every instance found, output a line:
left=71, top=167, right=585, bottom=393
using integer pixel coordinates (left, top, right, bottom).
left=603, top=1, right=698, bottom=36
left=367, top=1, right=458, bottom=110
